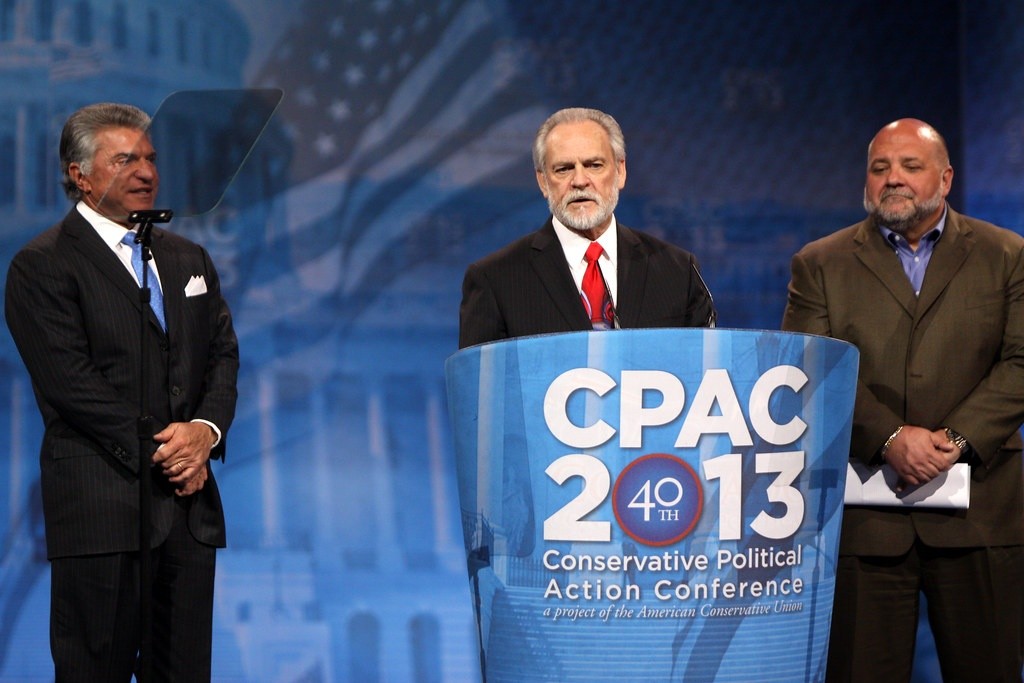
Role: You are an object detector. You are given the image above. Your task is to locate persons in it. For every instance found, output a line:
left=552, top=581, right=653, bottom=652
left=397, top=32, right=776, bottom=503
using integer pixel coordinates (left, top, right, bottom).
left=4, top=101, right=242, bottom=683
left=779, top=116, right=1024, bottom=683
left=456, top=105, right=715, bottom=351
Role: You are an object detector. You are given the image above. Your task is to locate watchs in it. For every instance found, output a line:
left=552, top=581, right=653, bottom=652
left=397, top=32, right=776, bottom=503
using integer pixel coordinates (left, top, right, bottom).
left=945, top=427, right=970, bottom=455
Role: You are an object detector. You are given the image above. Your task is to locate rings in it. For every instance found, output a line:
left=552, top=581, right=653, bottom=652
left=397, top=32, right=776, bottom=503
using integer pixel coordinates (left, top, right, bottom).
left=177, top=463, right=184, bottom=471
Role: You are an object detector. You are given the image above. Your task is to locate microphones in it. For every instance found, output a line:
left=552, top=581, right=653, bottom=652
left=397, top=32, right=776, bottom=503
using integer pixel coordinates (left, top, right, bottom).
left=692, top=264, right=715, bottom=330
left=596, top=260, right=620, bottom=330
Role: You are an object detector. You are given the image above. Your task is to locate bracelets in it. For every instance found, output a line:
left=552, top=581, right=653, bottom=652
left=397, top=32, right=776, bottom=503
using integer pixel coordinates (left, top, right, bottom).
left=879, top=424, right=903, bottom=464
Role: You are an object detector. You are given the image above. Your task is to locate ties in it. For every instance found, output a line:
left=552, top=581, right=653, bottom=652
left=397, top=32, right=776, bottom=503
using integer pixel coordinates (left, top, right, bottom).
left=121, top=231, right=166, bottom=333
left=579, top=242, right=615, bottom=330
